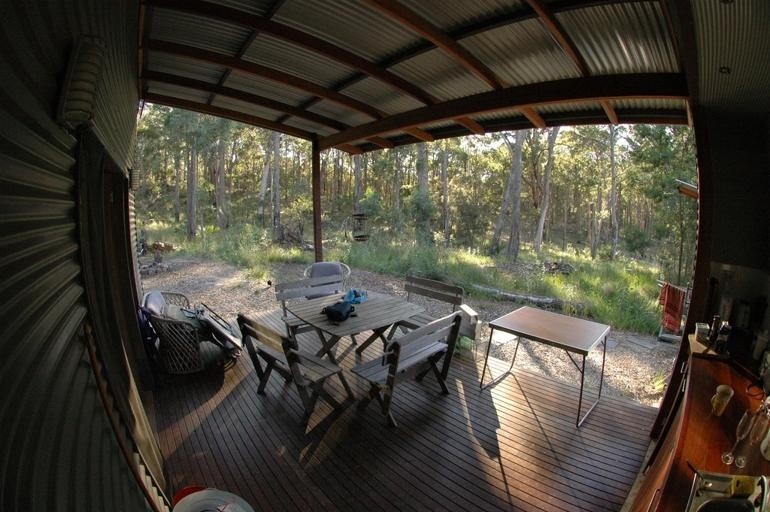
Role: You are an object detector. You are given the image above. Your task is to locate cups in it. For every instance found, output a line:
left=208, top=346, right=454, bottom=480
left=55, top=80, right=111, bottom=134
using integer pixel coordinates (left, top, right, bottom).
left=695, top=322, right=710, bottom=342
left=711, top=385, right=734, bottom=417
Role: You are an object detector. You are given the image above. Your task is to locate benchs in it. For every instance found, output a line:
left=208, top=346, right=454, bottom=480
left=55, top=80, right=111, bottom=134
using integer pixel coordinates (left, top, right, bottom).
left=274, top=274, right=359, bottom=350
left=348, top=310, right=463, bottom=429
left=235, top=312, right=344, bottom=428
left=384, top=274, right=467, bottom=372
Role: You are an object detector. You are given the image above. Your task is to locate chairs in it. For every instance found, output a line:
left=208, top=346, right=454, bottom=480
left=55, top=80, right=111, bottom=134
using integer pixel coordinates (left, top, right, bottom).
left=137, top=289, right=225, bottom=387
left=301, top=262, right=350, bottom=300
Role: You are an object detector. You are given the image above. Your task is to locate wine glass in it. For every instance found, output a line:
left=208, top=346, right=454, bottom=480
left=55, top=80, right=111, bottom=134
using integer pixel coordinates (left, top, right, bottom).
left=722, top=409, right=757, bottom=465
left=735, top=413, right=769, bottom=468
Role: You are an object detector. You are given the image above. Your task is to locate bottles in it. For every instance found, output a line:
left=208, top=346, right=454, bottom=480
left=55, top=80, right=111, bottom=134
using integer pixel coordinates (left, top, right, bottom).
left=715, top=327, right=729, bottom=354
left=709, top=315, right=721, bottom=347
left=753, top=330, right=768, bottom=360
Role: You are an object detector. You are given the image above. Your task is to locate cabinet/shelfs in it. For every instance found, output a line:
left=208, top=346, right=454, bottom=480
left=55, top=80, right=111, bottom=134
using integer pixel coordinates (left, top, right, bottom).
left=627, top=332, right=770, bottom=512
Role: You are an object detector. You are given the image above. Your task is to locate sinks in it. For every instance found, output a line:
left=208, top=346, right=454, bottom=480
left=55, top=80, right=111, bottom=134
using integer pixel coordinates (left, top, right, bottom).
left=685, top=471, right=769, bottom=512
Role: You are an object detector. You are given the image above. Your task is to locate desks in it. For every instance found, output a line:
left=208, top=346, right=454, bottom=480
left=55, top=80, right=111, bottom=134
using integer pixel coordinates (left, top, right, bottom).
left=478, top=304, right=612, bottom=429
left=283, top=288, right=427, bottom=402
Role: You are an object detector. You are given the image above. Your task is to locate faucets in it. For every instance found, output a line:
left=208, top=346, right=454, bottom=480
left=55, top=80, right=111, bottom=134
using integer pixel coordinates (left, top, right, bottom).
left=757, top=475, right=768, bottom=512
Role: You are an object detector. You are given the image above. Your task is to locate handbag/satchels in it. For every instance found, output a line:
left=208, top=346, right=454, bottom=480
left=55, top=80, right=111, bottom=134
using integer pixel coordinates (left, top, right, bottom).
left=160, top=303, right=207, bottom=342
left=321, top=301, right=355, bottom=325
